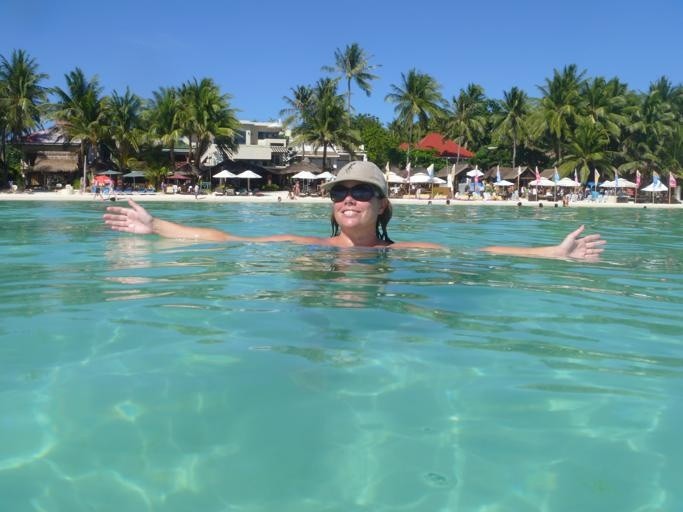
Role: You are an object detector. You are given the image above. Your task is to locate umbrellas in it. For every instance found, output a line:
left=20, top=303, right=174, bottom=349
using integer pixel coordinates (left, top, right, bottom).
left=305, top=170, right=317, bottom=191
left=121, top=172, right=147, bottom=190
left=529, top=176, right=558, bottom=189
left=429, top=176, right=448, bottom=195
left=555, top=176, right=581, bottom=188
left=236, top=170, right=262, bottom=191
left=491, top=180, right=514, bottom=196
left=97, top=169, right=123, bottom=186
left=599, top=179, right=613, bottom=188
left=608, top=178, right=638, bottom=194
left=640, top=180, right=668, bottom=204
left=90, top=174, right=115, bottom=190
left=211, top=169, right=237, bottom=183
left=313, top=171, right=337, bottom=192
left=291, top=171, right=312, bottom=191
left=382, top=171, right=409, bottom=197
left=403, top=173, right=434, bottom=194
left=165, top=173, right=193, bottom=187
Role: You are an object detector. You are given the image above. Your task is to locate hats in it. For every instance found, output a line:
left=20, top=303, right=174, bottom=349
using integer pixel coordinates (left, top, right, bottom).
left=318, top=160, right=388, bottom=198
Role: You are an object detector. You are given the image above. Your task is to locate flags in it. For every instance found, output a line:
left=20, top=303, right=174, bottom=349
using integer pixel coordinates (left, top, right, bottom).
left=636, top=169, right=641, bottom=188
left=426, top=162, right=434, bottom=185
left=614, top=169, right=619, bottom=187
left=449, top=164, right=454, bottom=192
left=404, top=162, right=410, bottom=181
left=385, top=161, right=390, bottom=177
left=474, top=165, right=479, bottom=192
left=669, top=171, right=677, bottom=188
left=535, top=165, right=540, bottom=185
left=594, top=168, right=600, bottom=188
left=496, top=165, right=500, bottom=183
left=574, top=168, right=578, bottom=186
left=652, top=170, right=661, bottom=188
left=553, top=166, right=560, bottom=184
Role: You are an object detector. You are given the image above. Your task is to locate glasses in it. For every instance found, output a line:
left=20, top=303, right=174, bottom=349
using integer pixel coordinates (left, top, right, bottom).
left=330, top=185, right=379, bottom=201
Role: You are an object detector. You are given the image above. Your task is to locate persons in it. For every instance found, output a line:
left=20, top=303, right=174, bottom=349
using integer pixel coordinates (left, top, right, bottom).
left=316, top=179, right=324, bottom=196
left=516, top=201, right=522, bottom=208
left=103, top=182, right=117, bottom=201
left=426, top=200, right=432, bottom=207
left=194, top=183, right=199, bottom=200
left=89, top=177, right=103, bottom=202
left=97, top=159, right=607, bottom=265
left=292, top=180, right=299, bottom=196
left=287, top=190, right=295, bottom=200
left=102, top=182, right=110, bottom=197
left=554, top=203, right=559, bottom=208
left=444, top=200, right=450, bottom=206
left=537, top=202, right=543, bottom=209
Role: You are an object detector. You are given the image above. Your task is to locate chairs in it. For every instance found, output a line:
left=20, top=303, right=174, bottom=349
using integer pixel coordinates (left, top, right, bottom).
left=26, top=184, right=48, bottom=191
left=214, top=187, right=265, bottom=197
left=91, top=186, right=157, bottom=202
